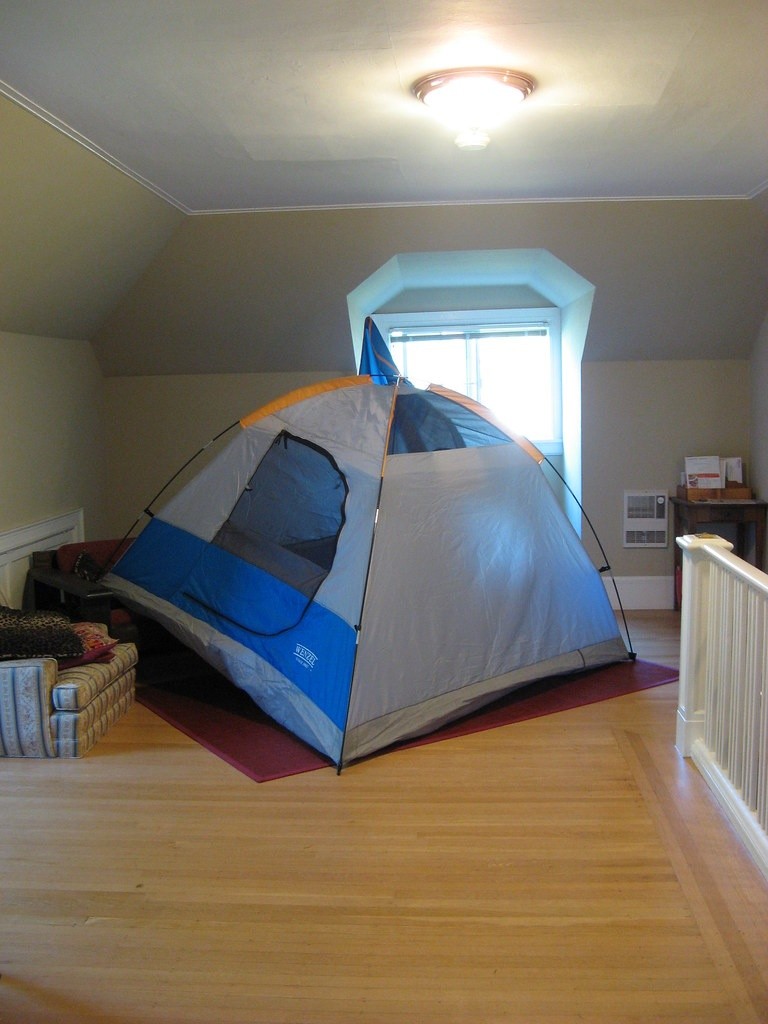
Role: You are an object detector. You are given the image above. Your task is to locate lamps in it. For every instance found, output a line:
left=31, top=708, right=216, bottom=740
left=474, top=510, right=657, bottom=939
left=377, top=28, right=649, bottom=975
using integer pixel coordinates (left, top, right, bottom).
left=409, top=66, right=538, bottom=117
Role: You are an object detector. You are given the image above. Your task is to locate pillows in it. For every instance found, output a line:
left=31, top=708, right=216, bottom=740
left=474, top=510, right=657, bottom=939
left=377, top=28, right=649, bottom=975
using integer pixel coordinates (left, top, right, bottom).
left=92, top=623, right=116, bottom=665
left=0, top=603, right=85, bottom=660
left=57, top=621, right=120, bottom=671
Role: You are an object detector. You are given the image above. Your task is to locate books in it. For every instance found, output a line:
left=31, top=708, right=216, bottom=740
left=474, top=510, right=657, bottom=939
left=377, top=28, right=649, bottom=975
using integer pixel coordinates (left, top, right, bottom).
left=685, top=456, right=743, bottom=489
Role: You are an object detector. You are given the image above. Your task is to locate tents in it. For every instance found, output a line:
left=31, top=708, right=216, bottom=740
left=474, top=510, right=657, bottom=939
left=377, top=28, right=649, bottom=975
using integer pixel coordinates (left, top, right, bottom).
left=92, top=316, right=637, bottom=775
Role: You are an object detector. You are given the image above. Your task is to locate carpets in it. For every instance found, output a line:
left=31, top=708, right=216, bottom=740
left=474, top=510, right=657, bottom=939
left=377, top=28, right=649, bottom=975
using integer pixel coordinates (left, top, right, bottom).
left=58, top=536, right=680, bottom=782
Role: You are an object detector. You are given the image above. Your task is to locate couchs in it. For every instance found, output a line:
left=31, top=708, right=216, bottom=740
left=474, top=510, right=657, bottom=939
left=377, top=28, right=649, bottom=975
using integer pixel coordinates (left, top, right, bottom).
left=0, top=643, right=137, bottom=759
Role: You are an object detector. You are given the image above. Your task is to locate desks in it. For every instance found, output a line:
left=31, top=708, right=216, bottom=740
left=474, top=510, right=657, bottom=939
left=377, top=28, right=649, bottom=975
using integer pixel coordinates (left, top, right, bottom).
left=669, top=496, right=768, bottom=610
